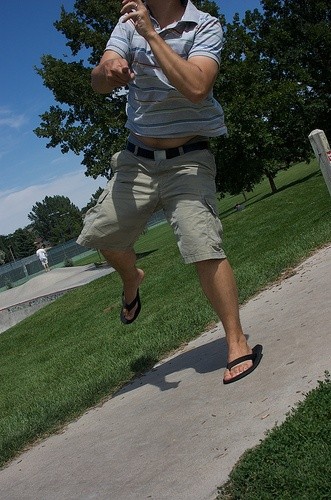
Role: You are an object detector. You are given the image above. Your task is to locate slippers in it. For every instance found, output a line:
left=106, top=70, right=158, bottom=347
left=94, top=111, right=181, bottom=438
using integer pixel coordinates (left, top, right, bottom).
left=120, top=287, right=141, bottom=324
left=223, top=344, right=263, bottom=384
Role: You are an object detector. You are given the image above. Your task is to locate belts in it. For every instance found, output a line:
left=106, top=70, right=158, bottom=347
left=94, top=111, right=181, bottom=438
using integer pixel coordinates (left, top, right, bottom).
left=126, top=140, right=211, bottom=161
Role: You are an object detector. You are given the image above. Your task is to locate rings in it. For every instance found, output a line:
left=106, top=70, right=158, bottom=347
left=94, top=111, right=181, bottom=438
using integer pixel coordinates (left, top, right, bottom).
left=134, top=4, right=138, bottom=9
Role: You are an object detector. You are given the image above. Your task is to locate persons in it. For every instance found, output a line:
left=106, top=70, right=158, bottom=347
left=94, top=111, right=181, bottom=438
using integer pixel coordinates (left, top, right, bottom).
left=36, top=245, right=51, bottom=272
left=76, top=0, right=263, bottom=386
